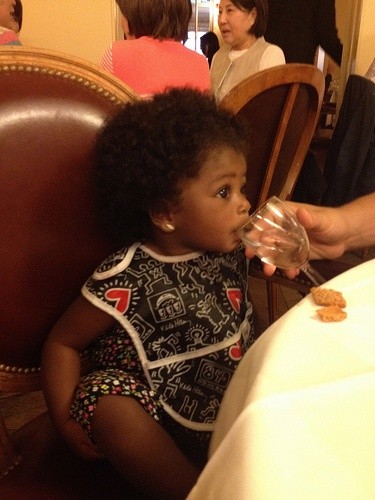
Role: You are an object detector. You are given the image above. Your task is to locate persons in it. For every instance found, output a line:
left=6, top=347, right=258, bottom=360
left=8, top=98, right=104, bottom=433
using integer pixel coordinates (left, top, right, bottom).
left=38, top=87, right=259, bottom=500
left=0, top=0, right=23, bottom=47
left=96, top=0, right=212, bottom=100
left=200, top=31, right=220, bottom=71
left=209, top=0, right=286, bottom=106
left=264, top=0, right=346, bottom=69
left=245, top=191, right=375, bottom=278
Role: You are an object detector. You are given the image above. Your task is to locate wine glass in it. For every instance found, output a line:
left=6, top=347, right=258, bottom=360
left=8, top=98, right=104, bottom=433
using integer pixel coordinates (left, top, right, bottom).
left=233, top=196, right=327, bottom=287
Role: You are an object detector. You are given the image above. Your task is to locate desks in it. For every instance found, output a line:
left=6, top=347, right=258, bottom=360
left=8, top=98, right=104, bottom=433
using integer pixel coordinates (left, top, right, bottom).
left=188, top=259, right=375, bottom=500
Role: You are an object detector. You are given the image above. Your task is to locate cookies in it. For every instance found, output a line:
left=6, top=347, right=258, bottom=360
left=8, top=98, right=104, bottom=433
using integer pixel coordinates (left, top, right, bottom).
left=310, top=287, right=346, bottom=308
left=316, top=305, right=347, bottom=322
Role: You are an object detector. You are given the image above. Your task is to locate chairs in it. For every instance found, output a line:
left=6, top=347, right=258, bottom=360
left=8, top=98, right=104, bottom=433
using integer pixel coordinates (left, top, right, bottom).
left=0, top=45, right=145, bottom=500
left=219, top=65, right=327, bottom=322
left=248, top=247, right=375, bottom=324
